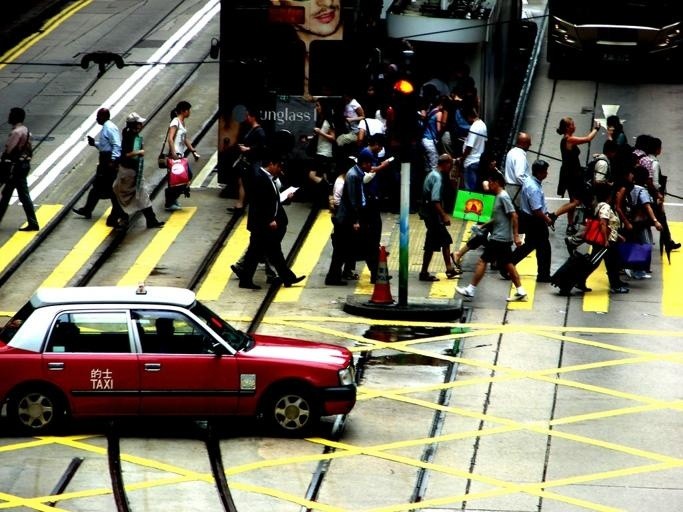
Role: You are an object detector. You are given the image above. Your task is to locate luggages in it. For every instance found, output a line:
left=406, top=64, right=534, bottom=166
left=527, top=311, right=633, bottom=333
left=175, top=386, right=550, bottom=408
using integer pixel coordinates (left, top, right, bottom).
left=551, top=247, right=609, bottom=295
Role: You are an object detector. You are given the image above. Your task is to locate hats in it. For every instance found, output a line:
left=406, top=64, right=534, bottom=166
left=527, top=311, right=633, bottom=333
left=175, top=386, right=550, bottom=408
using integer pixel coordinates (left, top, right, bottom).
left=358, top=149, right=379, bottom=163
left=125, top=112, right=147, bottom=123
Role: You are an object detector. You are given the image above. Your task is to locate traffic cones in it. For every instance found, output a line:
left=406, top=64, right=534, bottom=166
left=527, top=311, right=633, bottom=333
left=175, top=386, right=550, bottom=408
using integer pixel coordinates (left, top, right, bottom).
left=368, top=245, right=396, bottom=307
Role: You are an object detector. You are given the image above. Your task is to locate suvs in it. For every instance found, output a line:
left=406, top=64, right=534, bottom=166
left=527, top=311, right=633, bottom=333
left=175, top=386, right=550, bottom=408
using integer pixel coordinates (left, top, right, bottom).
left=1, top=280, right=357, bottom=438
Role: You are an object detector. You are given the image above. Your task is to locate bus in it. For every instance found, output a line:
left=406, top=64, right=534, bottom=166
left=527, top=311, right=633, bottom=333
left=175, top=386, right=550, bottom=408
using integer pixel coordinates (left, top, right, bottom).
left=208, top=1, right=384, bottom=199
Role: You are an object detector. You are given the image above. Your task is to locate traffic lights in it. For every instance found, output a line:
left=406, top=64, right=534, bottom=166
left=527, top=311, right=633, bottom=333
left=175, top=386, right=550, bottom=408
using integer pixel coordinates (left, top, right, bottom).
left=392, top=47, right=418, bottom=115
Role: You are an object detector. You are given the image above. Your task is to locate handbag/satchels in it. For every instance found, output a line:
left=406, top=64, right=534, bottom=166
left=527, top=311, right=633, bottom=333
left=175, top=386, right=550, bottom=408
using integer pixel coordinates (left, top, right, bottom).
left=648, top=176, right=667, bottom=198
left=617, top=242, right=651, bottom=265
left=158, top=154, right=168, bottom=168
left=630, top=205, right=659, bottom=224
left=582, top=218, right=611, bottom=247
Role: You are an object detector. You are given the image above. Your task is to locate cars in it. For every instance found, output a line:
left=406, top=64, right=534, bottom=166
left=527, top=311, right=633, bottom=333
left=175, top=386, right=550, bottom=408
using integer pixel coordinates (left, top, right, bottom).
left=544, top=0, right=681, bottom=76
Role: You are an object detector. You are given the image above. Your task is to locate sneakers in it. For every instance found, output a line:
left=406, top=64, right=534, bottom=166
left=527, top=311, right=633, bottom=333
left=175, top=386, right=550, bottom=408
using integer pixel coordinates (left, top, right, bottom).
left=575, top=268, right=652, bottom=294
left=18, top=202, right=246, bottom=231
left=665, top=241, right=681, bottom=251
left=419, top=213, right=578, bottom=303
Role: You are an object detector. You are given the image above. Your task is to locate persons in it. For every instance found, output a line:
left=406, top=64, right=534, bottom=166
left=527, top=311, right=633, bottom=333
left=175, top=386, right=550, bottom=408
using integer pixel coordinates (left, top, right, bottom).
left=225, top=106, right=306, bottom=289
left=72, top=108, right=165, bottom=229
left=307, top=80, right=487, bottom=285
left=155, top=317, right=175, bottom=342
left=163, top=101, right=199, bottom=210
left=264, top=0, right=345, bottom=95
left=0, top=107, right=40, bottom=231
left=450, top=114, right=682, bottom=301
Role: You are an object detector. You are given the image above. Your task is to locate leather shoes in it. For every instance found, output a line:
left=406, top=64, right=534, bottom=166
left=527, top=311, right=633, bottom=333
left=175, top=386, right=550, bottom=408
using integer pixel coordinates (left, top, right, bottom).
left=230, top=262, right=393, bottom=289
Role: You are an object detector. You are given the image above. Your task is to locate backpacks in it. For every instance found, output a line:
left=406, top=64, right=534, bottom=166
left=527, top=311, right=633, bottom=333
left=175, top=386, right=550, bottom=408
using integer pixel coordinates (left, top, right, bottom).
left=586, top=159, right=608, bottom=180
